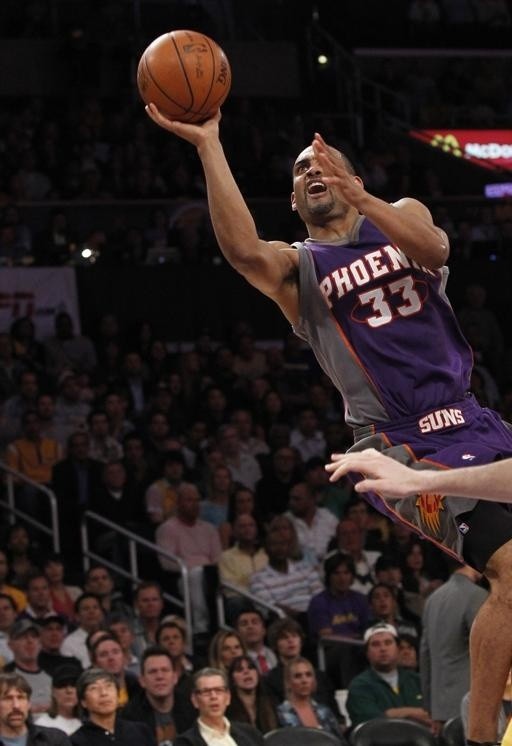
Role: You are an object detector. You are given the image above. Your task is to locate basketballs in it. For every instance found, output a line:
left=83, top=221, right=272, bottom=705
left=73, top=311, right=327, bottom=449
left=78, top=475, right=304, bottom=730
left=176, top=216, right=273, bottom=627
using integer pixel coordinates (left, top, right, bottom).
left=136, top=29, right=231, bottom=125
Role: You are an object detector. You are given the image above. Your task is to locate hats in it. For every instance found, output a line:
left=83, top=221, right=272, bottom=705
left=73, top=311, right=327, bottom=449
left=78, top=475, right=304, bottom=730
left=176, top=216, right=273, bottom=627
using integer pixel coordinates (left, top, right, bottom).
left=51, top=665, right=83, bottom=686
left=10, top=621, right=42, bottom=637
left=41, top=611, right=67, bottom=626
left=362, top=621, right=400, bottom=646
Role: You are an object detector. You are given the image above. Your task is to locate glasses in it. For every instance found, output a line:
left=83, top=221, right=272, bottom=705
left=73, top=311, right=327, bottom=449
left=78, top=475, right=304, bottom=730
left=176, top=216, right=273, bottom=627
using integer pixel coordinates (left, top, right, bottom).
left=197, top=686, right=227, bottom=695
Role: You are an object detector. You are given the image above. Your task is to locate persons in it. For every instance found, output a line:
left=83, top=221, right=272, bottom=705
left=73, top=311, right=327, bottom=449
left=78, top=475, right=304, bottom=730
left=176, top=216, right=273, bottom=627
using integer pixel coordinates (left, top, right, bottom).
left=0, top=0, right=510, bottom=745
